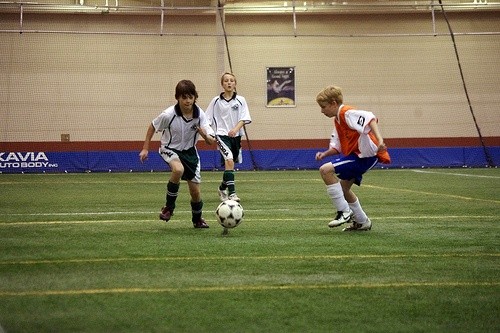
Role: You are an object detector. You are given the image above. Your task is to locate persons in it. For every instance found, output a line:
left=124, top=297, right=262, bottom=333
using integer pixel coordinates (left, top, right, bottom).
left=315, top=87, right=392, bottom=230
left=205, top=72, right=252, bottom=201
left=139, top=80, right=215, bottom=229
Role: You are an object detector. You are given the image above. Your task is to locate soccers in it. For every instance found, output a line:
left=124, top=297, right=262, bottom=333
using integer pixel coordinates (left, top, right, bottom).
left=216, top=200, right=244, bottom=228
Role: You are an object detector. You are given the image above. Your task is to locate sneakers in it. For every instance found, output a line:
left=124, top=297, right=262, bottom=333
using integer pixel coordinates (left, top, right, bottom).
left=342, top=218, right=372, bottom=231
left=193, top=218, right=209, bottom=228
left=160, top=207, right=174, bottom=221
left=328, top=209, right=355, bottom=227
left=218, top=187, right=229, bottom=201
left=228, top=193, right=240, bottom=201
